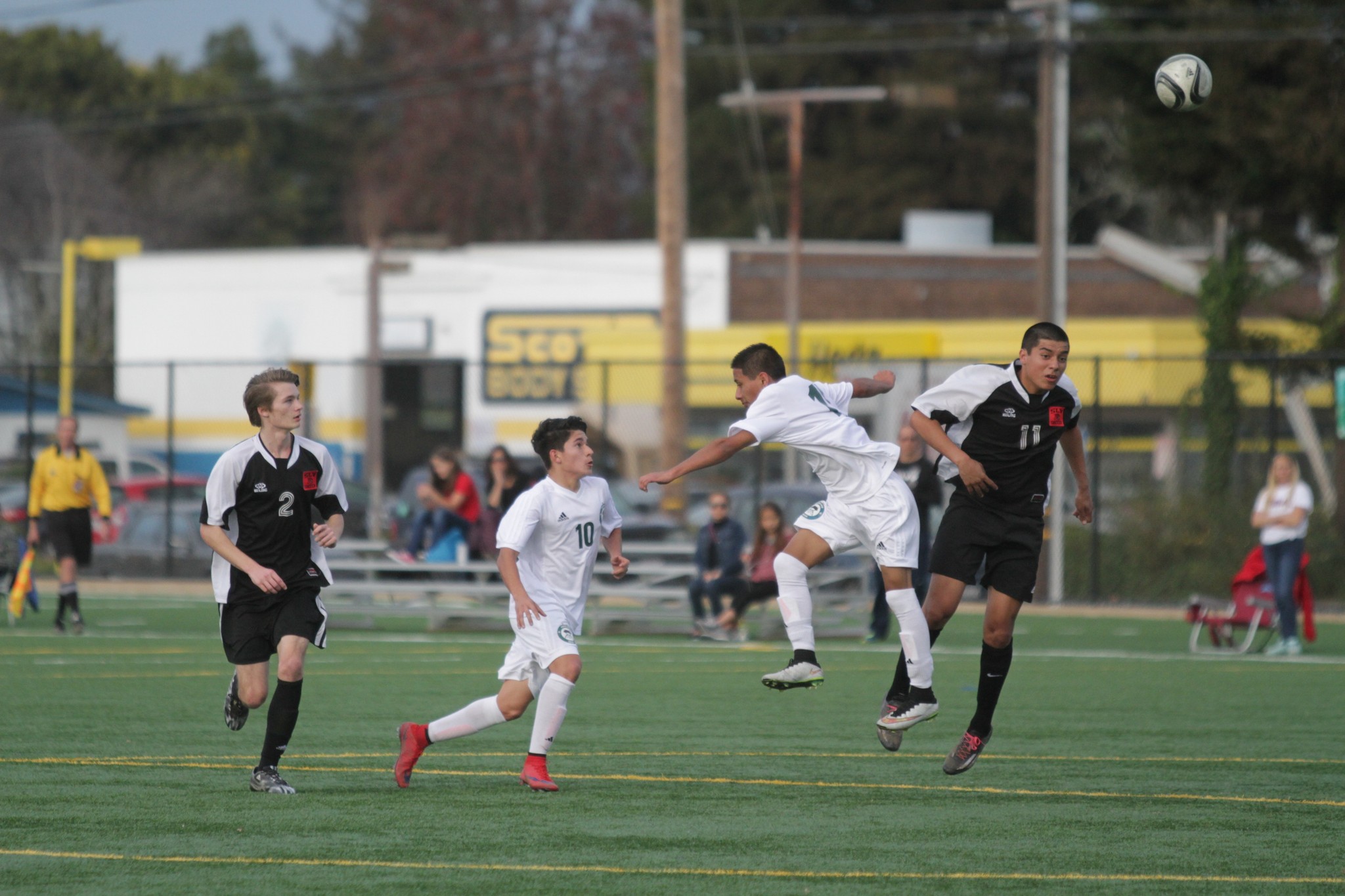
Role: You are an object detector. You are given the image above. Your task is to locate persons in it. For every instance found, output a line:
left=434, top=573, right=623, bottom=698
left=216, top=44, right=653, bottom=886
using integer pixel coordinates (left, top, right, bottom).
left=638, top=342, right=946, bottom=730
left=26, top=412, right=114, bottom=633
left=1249, top=450, right=1314, bottom=655
left=378, top=417, right=629, bottom=791
left=876, top=321, right=1093, bottom=775
left=199, top=369, right=348, bottom=793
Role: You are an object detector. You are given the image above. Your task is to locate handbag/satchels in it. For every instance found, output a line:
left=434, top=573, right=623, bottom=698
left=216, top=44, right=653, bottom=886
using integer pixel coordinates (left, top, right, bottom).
left=424, top=527, right=469, bottom=563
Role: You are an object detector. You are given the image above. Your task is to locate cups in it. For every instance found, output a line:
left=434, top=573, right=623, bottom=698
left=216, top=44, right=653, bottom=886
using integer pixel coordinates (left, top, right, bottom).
left=456, top=543, right=469, bottom=565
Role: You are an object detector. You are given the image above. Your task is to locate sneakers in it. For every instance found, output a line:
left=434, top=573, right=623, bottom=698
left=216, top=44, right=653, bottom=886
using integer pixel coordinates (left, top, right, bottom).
left=761, top=658, right=825, bottom=691
left=250, top=765, right=296, bottom=794
left=392, top=722, right=423, bottom=789
left=877, top=688, right=903, bottom=752
left=520, top=758, right=559, bottom=793
left=942, top=723, right=993, bottom=776
left=877, top=693, right=939, bottom=730
left=224, top=667, right=249, bottom=731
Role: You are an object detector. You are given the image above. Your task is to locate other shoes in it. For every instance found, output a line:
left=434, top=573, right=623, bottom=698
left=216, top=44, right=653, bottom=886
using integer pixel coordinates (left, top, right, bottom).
left=1286, top=635, right=1303, bottom=656
left=858, top=633, right=889, bottom=646
left=53, top=618, right=67, bottom=633
left=1266, top=639, right=1286, bottom=657
left=386, top=549, right=416, bottom=565
left=695, top=617, right=721, bottom=631
left=70, top=612, right=84, bottom=634
left=699, top=630, right=731, bottom=643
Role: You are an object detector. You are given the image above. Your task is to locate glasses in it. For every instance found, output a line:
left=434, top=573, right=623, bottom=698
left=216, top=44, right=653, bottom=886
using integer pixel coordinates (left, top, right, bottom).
left=709, top=502, right=728, bottom=511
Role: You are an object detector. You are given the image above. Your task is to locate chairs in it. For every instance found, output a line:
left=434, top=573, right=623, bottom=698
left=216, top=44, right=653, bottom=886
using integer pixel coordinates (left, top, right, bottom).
left=1189, top=545, right=1310, bottom=655
